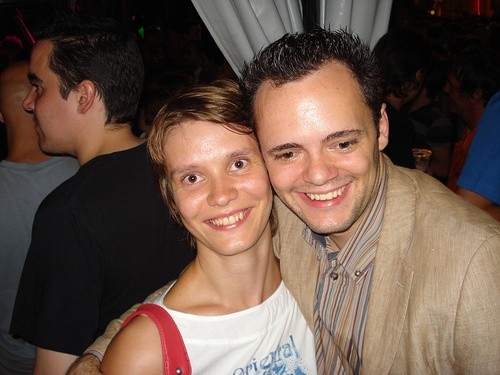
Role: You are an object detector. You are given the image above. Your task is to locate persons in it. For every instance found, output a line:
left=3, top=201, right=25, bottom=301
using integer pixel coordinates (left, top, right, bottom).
left=0, top=0, right=500, bottom=222
left=8, top=24, right=196, bottom=375
left=1, top=66, right=78, bottom=375
left=64, top=27, right=500, bottom=375
left=100, top=79, right=316, bottom=375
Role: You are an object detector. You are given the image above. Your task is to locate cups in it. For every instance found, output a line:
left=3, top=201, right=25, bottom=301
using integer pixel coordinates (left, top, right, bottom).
left=413, top=148, right=433, bottom=171
